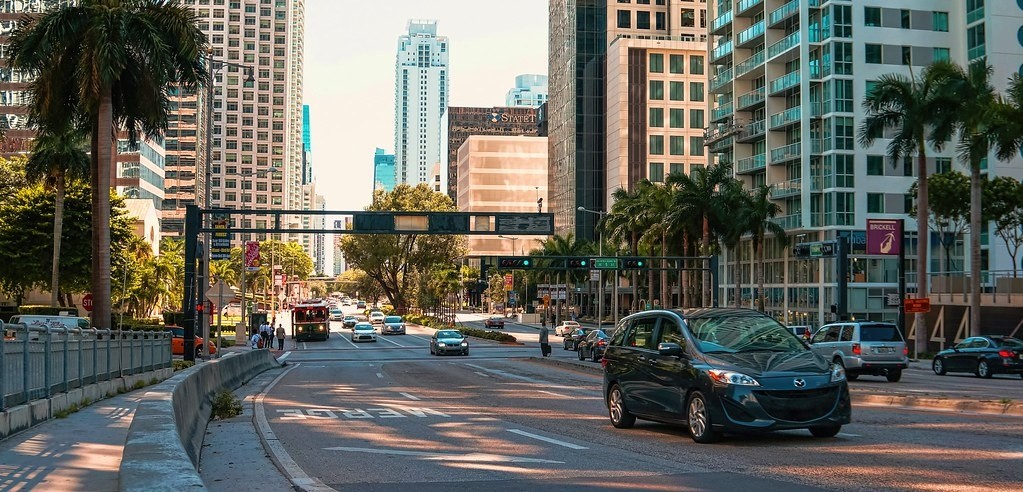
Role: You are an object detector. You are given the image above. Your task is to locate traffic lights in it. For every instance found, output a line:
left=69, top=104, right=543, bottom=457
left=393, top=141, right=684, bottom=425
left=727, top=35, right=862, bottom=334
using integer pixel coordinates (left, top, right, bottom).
left=567, top=258, right=591, bottom=269
left=497, top=257, right=533, bottom=269
left=624, top=258, right=644, bottom=269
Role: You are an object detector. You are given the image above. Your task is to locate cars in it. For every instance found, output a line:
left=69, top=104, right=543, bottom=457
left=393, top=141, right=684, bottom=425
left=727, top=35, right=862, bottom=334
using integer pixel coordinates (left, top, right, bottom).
left=325, top=291, right=339, bottom=313
left=330, top=309, right=344, bottom=321
left=563, top=328, right=596, bottom=351
left=430, top=329, right=469, bottom=356
left=555, top=321, right=580, bottom=337
left=485, top=316, right=504, bottom=329
left=338, top=295, right=359, bottom=306
left=351, top=322, right=377, bottom=342
left=368, top=311, right=385, bottom=324
left=577, top=328, right=616, bottom=361
left=932, top=335, right=1023, bottom=378
left=341, top=315, right=360, bottom=328
left=158, top=324, right=216, bottom=357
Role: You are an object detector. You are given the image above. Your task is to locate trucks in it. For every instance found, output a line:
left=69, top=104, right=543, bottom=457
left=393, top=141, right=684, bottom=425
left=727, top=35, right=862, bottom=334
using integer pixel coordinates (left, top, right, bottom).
left=7, top=311, right=91, bottom=340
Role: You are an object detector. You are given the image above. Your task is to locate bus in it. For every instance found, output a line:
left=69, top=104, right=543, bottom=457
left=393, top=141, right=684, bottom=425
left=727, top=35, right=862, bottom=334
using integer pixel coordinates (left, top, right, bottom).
left=291, top=299, right=330, bottom=340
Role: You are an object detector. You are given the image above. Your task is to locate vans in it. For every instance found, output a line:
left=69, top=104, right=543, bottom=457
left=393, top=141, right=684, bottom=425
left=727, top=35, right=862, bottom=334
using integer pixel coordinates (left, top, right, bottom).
left=381, top=316, right=405, bottom=335
left=761, top=325, right=810, bottom=344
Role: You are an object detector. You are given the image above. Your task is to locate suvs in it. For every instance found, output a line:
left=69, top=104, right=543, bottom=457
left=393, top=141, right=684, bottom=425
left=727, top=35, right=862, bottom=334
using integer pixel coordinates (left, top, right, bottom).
left=806, top=320, right=908, bottom=382
left=357, top=301, right=366, bottom=309
left=602, top=307, right=851, bottom=442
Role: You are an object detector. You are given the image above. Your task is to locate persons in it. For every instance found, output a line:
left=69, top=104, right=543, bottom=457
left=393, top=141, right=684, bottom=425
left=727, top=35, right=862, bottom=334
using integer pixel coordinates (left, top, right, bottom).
left=804, top=327, right=810, bottom=338
left=276, top=324, right=286, bottom=350
left=571, top=311, right=576, bottom=321
left=251, top=321, right=275, bottom=348
left=550, top=314, right=556, bottom=328
left=538, top=322, right=548, bottom=357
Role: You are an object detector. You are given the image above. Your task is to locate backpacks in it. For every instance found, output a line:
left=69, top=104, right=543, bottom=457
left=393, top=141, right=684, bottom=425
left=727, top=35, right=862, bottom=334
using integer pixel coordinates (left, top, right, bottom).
left=253, top=335, right=263, bottom=349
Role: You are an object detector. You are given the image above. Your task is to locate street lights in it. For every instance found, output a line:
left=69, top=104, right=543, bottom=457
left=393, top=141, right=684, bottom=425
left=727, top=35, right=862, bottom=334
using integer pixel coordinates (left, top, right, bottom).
left=577, top=206, right=607, bottom=329
left=231, top=168, right=276, bottom=319
